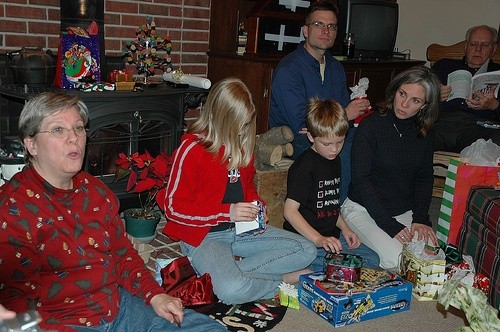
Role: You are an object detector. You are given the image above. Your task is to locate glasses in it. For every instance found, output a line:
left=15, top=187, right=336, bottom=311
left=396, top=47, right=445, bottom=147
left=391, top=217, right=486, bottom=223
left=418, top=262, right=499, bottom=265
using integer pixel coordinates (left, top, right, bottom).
left=37, top=123, right=91, bottom=138
left=306, top=21, right=338, bottom=31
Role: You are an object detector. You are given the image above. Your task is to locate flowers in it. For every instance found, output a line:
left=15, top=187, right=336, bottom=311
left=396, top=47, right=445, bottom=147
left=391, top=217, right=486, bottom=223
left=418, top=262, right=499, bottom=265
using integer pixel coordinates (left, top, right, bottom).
left=115, top=150, right=171, bottom=216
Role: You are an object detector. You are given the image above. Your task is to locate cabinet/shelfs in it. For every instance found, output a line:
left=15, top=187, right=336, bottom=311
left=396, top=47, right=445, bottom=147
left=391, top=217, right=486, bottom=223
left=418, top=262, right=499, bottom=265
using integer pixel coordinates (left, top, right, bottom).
left=1, top=83, right=209, bottom=200
left=207, top=0, right=414, bottom=135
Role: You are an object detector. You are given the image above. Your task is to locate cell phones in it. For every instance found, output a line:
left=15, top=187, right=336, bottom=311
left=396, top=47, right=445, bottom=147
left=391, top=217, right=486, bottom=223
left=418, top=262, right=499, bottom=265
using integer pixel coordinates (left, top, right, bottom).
left=0, top=309, right=43, bottom=332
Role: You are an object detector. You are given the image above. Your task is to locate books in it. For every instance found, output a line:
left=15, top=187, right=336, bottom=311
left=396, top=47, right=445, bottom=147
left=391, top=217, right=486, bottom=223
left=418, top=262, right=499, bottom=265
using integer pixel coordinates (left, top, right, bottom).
left=446, top=69, right=500, bottom=102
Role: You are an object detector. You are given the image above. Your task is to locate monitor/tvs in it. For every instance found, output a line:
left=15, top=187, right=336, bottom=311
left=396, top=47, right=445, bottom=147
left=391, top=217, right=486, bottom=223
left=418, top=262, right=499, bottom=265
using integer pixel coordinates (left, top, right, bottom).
left=328, top=0, right=399, bottom=61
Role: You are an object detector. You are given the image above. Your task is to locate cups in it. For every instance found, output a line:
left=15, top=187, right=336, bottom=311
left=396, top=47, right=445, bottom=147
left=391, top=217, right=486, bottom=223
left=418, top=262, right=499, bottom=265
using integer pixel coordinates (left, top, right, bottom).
left=342, top=33, right=354, bottom=61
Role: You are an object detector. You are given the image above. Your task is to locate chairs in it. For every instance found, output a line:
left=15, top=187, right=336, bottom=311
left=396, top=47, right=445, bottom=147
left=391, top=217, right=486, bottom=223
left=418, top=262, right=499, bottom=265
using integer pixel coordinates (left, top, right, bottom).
left=427, top=41, right=500, bottom=198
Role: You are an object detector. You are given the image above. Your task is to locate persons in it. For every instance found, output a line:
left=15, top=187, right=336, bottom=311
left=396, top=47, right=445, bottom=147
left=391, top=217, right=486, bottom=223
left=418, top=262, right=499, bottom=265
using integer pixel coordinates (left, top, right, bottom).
left=269, top=0, right=370, bottom=207
left=431, top=25, right=500, bottom=152
left=162, top=78, right=317, bottom=304
left=0, top=91, right=230, bottom=332
left=283, top=96, right=380, bottom=283
left=339, top=66, right=445, bottom=270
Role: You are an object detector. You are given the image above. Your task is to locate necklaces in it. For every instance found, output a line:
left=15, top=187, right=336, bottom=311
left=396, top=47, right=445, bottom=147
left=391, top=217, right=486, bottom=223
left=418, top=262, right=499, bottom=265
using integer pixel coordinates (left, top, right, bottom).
left=393, top=121, right=411, bottom=138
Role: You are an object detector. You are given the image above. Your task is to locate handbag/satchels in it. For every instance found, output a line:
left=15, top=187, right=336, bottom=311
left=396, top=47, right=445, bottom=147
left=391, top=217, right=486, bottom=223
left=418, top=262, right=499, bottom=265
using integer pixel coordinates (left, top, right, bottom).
left=397, top=244, right=446, bottom=301
left=436, top=156, right=500, bottom=248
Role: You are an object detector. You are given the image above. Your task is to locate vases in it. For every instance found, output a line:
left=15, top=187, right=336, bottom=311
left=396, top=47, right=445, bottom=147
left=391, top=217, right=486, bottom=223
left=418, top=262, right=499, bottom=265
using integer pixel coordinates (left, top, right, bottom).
left=124, top=208, right=161, bottom=244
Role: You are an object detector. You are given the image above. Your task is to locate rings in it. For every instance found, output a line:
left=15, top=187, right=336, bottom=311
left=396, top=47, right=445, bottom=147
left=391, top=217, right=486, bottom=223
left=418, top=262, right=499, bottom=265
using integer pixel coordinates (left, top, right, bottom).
left=475, top=105, right=477, bottom=108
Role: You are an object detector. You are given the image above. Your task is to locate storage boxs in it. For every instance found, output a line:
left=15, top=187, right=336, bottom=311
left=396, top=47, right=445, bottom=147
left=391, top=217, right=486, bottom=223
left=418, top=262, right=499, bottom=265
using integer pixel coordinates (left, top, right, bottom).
left=298, top=266, right=412, bottom=327
left=254, top=167, right=286, bottom=229
left=235, top=201, right=265, bottom=237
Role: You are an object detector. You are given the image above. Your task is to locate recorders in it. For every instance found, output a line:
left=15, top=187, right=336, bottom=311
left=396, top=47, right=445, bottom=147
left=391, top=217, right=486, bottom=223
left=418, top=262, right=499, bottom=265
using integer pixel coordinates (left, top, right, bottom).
left=245, top=16, right=304, bottom=58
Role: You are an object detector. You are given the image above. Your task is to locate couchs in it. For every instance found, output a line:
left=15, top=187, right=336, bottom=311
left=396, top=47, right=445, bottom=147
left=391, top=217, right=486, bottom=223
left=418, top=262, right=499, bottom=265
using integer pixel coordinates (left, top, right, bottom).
left=456, top=185, right=500, bottom=313
